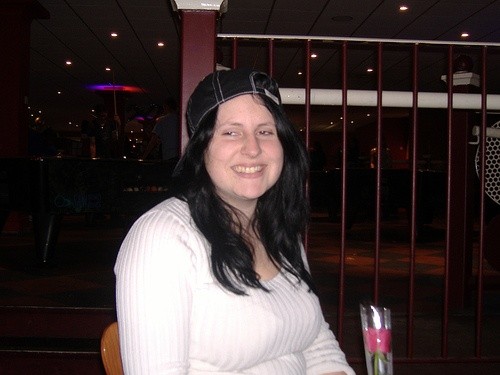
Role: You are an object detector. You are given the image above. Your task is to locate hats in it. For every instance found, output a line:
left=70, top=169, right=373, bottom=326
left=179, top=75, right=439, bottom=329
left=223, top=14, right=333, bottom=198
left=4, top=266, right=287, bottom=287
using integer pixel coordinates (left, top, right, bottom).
left=164, top=66, right=282, bottom=197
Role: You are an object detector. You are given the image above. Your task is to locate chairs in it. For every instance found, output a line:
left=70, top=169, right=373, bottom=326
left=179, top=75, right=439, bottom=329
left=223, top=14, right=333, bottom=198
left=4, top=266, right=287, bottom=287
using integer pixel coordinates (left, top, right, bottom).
left=101, top=322, right=124, bottom=375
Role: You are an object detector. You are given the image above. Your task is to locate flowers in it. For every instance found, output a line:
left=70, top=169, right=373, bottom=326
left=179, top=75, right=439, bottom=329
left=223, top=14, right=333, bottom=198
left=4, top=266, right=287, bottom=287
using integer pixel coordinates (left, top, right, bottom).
left=363, top=325, right=391, bottom=375
